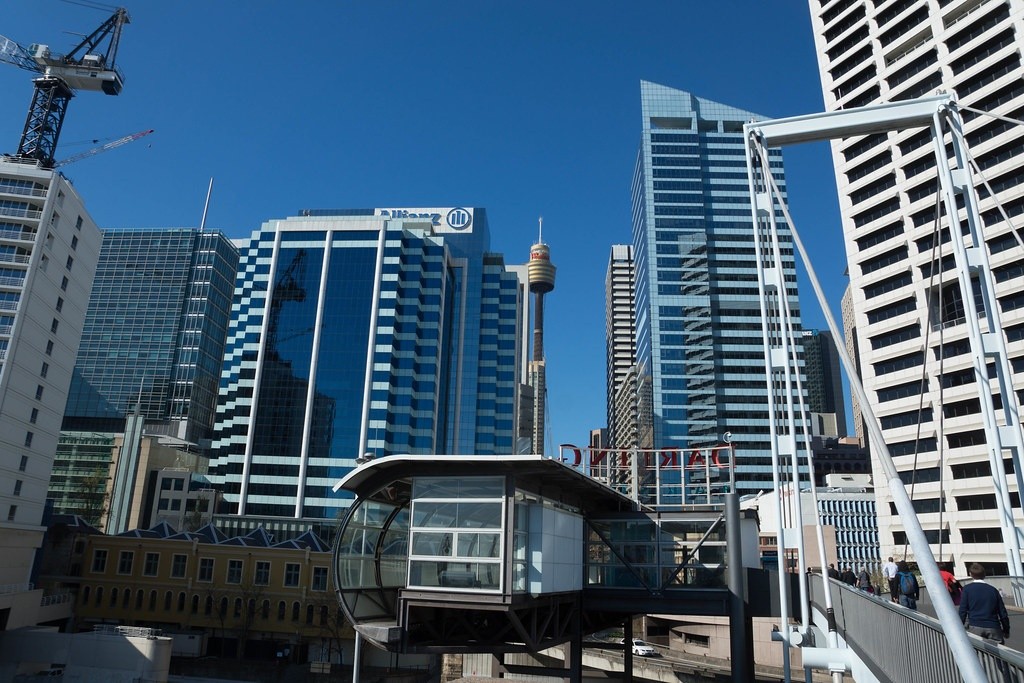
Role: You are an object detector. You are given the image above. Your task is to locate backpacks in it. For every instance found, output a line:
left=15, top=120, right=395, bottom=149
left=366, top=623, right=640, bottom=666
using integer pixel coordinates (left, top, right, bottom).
left=897, top=571, right=914, bottom=593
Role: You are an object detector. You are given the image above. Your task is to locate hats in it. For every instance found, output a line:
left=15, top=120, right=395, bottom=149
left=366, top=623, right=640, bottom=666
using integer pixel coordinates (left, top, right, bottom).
left=807, top=567, right=812, bottom=571
left=829, top=563, right=834, bottom=567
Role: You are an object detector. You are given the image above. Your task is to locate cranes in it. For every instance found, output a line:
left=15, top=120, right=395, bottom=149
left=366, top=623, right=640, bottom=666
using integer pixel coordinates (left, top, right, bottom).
left=0, top=0, right=134, bottom=165
left=48, top=127, right=156, bottom=169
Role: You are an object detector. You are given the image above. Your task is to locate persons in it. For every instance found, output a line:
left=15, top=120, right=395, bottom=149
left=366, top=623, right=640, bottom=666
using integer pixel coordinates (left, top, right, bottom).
left=938, top=564, right=963, bottom=605
left=828, top=564, right=856, bottom=586
left=856, top=567, right=870, bottom=591
left=806, top=567, right=811, bottom=574
left=893, top=560, right=920, bottom=610
left=882, top=557, right=899, bottom=604
left=868, top=580, right=881, bottom=596
left=958, top=564, right=1010, bottom=645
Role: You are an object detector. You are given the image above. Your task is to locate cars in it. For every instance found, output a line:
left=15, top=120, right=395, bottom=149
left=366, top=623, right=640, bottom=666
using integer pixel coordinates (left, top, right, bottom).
left=620, top=638, right=656, bottom=657
